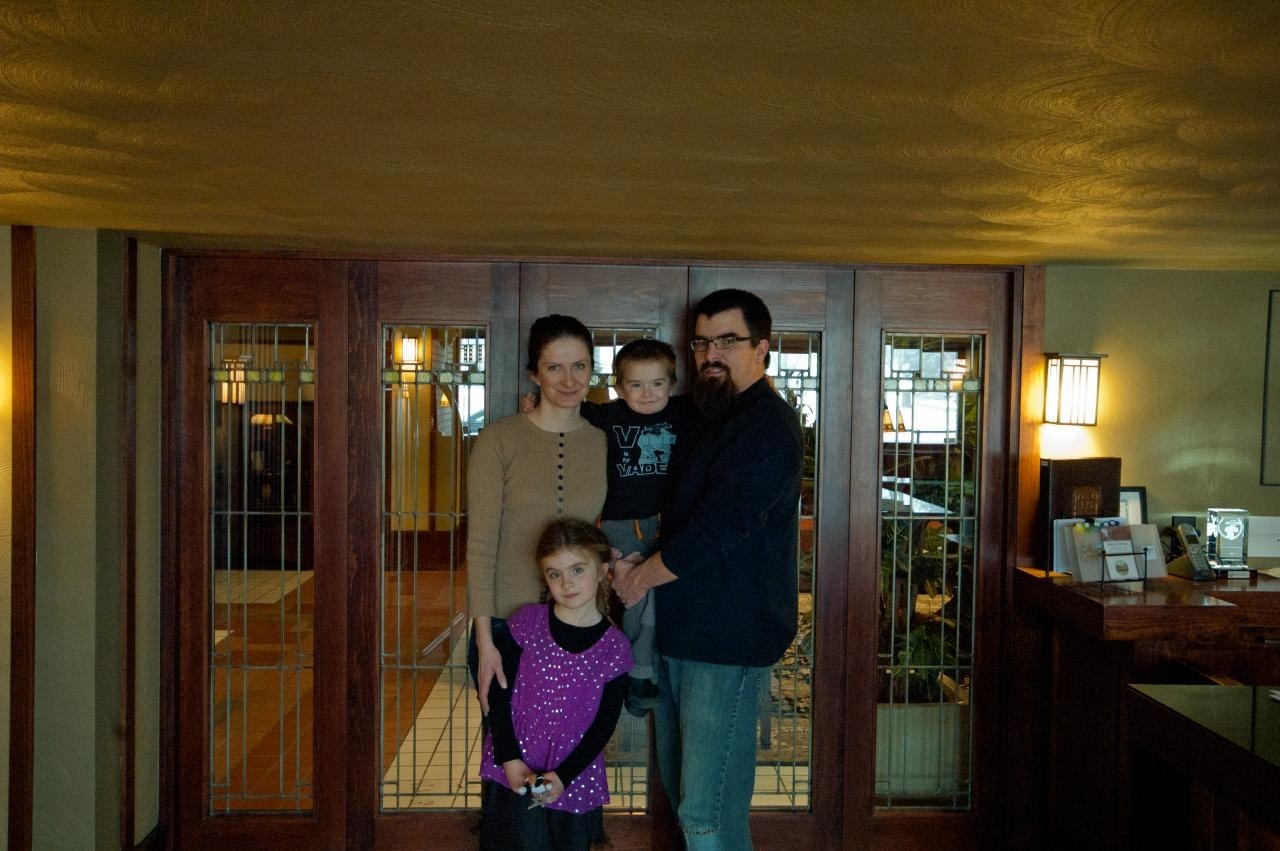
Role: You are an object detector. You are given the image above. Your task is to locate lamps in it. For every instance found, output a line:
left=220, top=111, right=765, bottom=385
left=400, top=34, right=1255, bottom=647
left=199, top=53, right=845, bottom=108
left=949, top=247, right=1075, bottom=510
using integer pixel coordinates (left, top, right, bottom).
left=883, top=398, right=895, bottom=432
left=222, top=358, right=244, bottom=404
left=1043, top=350, right=1108, bottom=426
left=400, top=334, right=419, bottom=361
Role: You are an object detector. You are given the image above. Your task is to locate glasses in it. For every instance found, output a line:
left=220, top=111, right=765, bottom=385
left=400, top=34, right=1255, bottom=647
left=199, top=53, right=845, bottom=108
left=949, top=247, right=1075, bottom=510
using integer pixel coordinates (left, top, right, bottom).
left=690, top=332, right=761, bottom=352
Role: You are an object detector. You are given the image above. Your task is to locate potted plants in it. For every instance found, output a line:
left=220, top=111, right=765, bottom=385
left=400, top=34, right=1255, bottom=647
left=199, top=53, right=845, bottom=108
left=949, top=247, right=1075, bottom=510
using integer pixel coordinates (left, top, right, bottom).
left=875, top=507, right=969, bottom=803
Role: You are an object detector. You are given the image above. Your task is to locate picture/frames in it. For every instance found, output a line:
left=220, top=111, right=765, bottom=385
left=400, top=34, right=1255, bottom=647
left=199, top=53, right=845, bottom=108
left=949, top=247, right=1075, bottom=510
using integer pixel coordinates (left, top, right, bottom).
left=1120, top=486, right=1148, bottom=526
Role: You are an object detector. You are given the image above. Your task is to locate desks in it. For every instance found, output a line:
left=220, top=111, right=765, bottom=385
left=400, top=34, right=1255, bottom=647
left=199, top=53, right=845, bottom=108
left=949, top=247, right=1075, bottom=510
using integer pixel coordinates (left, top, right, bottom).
left=1014, top=562, right=1280, bottom=851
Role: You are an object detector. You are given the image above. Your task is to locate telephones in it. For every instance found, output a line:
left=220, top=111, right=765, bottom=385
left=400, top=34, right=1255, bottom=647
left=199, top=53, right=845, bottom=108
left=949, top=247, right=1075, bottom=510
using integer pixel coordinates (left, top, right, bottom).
left=1166, top=524, right=1216, bottom=580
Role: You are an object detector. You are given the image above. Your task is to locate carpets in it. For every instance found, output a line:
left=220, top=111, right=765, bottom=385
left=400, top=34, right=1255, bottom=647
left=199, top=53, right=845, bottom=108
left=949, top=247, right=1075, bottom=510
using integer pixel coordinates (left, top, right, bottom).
left=601, top=594, right=808, bottom=767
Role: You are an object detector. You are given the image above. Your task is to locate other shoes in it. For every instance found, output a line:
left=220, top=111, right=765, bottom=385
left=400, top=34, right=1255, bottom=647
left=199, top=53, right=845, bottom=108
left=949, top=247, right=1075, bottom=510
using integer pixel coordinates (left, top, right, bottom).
left=623, top=671, right=658, bottom=718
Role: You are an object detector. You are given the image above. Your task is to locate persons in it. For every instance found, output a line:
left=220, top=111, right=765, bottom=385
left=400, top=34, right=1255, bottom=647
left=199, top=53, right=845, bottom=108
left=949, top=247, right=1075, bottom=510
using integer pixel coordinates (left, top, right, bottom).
left=478, top=519, right=633, bottom=851
left=466, top=316, right=608, bottom=851
left=519, top=337, right=688, bottom=719
left=610, top=287, right=805, bottom=851
